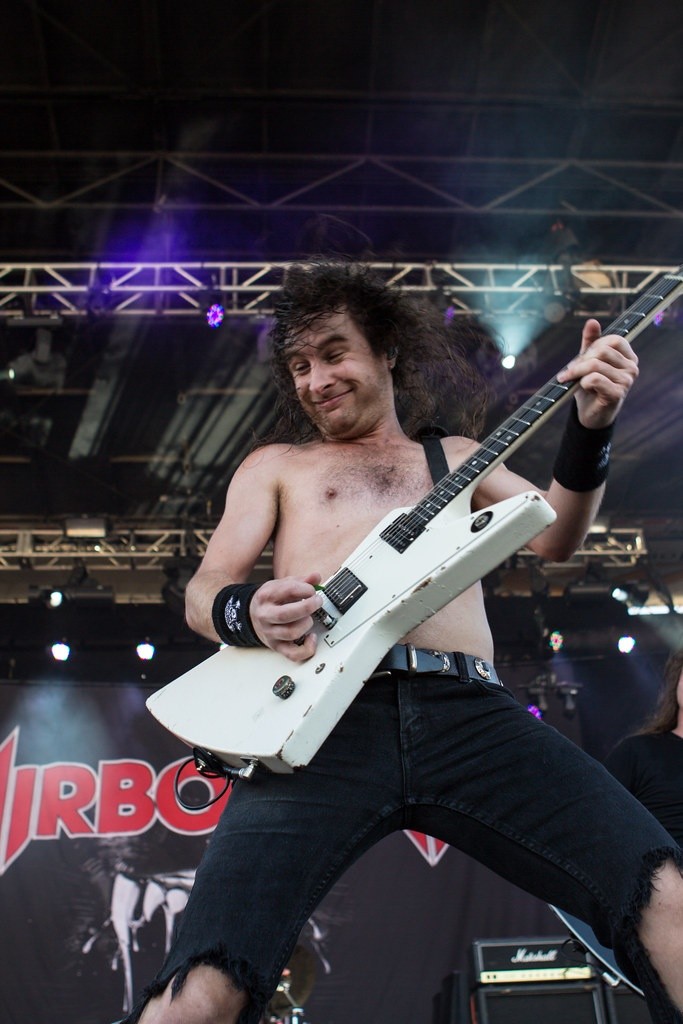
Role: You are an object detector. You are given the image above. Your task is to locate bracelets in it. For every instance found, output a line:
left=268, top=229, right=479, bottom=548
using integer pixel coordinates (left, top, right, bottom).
left=212, top=583, right=263, bottom=647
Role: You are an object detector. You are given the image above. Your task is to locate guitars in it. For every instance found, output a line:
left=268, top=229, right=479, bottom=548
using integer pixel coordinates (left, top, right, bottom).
left=143, top=268, right=683, bottom=776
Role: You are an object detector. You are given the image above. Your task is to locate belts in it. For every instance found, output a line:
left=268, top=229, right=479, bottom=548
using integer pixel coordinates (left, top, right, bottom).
left=379, top=644, right=503, bottom=686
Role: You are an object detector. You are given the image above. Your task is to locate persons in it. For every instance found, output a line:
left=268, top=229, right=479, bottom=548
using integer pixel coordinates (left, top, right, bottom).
left=601, top=647, right=683, bottom=853
left=122, top=263, right=683, bottom=1024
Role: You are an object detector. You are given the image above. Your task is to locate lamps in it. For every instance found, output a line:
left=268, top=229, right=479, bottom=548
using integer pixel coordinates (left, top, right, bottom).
left=38, top=588, right=64, bottom=609
left=485, top=555, right=649, bottom=653
left=0, top=410, right=52, bottom=447
left=136, top=635, right=163, bottom=661
left=198, top=288, right=226, bottom=328
left=46, top=640, right=72, bottom=664
